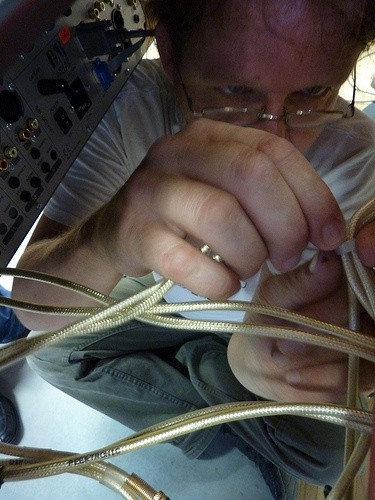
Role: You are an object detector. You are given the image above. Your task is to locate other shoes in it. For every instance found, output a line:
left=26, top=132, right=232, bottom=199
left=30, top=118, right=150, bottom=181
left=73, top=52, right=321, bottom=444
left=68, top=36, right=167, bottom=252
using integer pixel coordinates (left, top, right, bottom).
left=228, top=431, right=300, bottom=500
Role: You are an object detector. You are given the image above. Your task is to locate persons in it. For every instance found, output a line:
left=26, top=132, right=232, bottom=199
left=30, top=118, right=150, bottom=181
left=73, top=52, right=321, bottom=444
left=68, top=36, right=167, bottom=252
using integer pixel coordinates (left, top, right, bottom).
left=9, top=0, right=375, bottom=500
left=0, top=281, right=34, bottom=446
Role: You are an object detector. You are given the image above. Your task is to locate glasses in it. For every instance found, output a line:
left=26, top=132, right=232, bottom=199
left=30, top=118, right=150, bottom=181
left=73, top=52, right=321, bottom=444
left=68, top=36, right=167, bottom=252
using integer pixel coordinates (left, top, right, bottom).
left=166, top=22, right=356, bottom=128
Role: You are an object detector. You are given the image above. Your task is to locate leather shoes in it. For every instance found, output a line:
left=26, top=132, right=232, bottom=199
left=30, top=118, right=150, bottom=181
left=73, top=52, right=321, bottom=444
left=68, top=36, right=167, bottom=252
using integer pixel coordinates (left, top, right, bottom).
left=0, top=393, right=21, bottom=444
